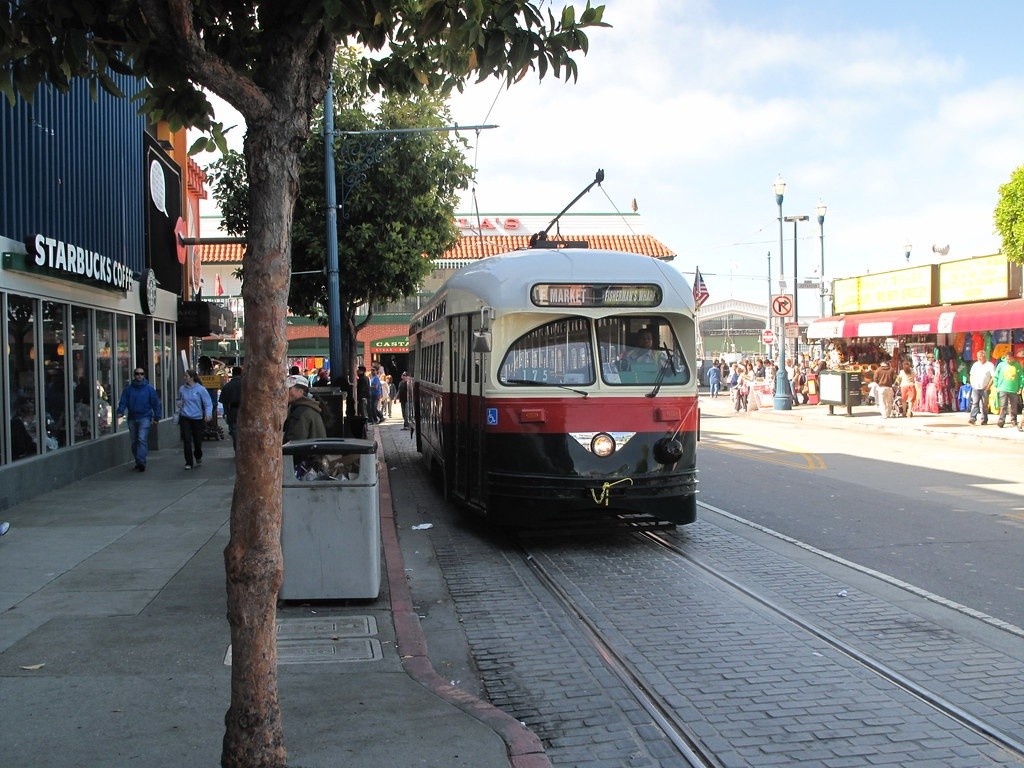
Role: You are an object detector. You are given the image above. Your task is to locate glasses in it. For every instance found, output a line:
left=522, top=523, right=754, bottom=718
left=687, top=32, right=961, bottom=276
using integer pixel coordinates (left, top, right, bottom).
left=135, top=372, right=145, bottom=375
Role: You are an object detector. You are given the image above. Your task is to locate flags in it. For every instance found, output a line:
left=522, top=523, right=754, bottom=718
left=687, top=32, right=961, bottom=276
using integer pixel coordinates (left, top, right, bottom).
left=215, top=274, right=224, bottom=296
left=692, top=269, right=709, bottom=309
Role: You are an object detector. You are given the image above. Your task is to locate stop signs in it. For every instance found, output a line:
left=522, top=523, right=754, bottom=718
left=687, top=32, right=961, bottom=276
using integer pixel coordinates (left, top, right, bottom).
left=762, top=330, right=774, bottom=344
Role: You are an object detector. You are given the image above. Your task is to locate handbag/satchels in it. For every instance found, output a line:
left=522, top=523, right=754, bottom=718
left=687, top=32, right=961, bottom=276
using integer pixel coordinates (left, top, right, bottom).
left=173, top=411, right=181, bottom=424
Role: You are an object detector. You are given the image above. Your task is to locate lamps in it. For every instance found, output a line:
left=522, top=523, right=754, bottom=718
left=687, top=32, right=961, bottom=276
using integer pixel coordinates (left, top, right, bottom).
left=102, top=342, right=110, bottom=358
left=56, top=338, right=64, bottom=356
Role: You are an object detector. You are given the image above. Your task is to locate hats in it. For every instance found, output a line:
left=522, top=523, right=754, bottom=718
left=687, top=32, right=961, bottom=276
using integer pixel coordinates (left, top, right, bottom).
left=287, top=375, right=308, bottom=388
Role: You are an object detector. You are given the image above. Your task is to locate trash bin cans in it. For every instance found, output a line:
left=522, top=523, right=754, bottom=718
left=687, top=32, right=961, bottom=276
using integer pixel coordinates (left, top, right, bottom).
left=308, top=387, right=343, bottom=436
left=344, top=416, right=368, bottom=438
left=279, top=438, right=380, bottom=606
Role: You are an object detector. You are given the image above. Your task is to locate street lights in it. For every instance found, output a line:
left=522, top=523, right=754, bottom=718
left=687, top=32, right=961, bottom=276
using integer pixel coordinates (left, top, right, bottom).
left=816, top=197, right=827, bottom=359
left=772, top=172, right=792, bottom=410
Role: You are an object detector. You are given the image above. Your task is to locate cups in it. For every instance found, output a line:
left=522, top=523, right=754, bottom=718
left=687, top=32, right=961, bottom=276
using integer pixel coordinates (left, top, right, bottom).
left=302, top=468, right=320, bottom=481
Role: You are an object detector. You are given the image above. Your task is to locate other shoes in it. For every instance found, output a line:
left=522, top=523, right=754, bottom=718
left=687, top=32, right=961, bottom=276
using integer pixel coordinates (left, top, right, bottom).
left=1012, top=420, right=1016, bottom=425
left=185, top=465, right=192, bottom=469
left=135, top=464, right=145, bottom=473
left=369, top=420, right=378, bottom=425
left=400, top=427, right=409, bottom=430
left=981, top=421, right=987, bottom=424
left=969, top=420, right=975, bottom=425
left=379, top=418, right=385, bottom=423
left=194, top=451, right=202, bottom=464
left=998, top=422, right=1004, bottom=428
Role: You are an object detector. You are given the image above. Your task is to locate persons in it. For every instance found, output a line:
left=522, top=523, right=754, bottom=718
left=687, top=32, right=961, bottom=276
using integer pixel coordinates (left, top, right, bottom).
left=219, top=367, right=242, bottom=457
left=627, top=329, right=668, bottom=369
left=707, top=359, right=730, bottom=398
left=282, top=366, right=331, bottom=444
left=357, top=365, right=411, bottom=430
left=177, top=370, right=213, bottom=470
left=968, top=350, right=995, bottom=425
left=874, top=359, right=894, bottom=419
left=730, top=351, right=829, bottom=412
left=865, top=382, right=879, bottom=405
left=117, top=368, right=162, bottom=472
left=894, top=360, right=916, bottom=417
left=994, top=352, right=1024, bottom=432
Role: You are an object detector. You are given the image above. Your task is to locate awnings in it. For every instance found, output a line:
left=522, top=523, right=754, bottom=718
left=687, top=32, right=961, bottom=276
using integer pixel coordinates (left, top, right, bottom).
left=807, top=298, right=1024, bottom=338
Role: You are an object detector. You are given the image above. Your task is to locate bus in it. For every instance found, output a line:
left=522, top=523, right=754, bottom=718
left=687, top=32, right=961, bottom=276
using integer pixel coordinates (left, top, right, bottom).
left=407, top=169, right=700, bottom=540
